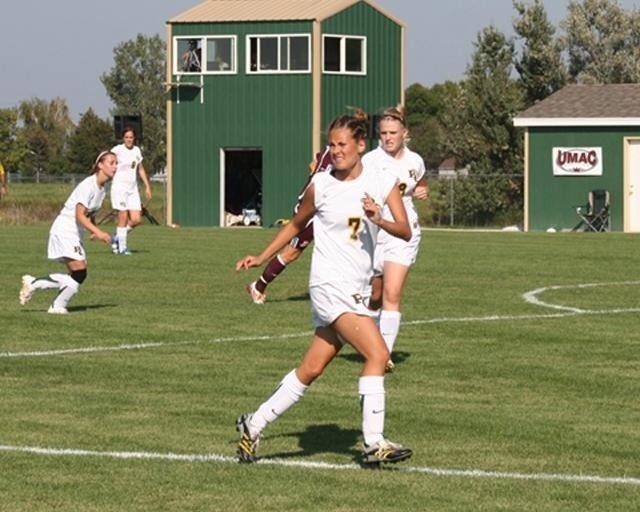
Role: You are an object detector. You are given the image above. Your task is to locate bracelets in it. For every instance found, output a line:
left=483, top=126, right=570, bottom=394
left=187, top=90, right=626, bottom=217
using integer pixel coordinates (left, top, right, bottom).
left=1, top=184, right=6, bottom=188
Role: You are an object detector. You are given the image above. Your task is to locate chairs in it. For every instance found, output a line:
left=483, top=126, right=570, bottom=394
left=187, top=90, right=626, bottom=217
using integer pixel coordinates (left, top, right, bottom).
left=577, top=189, right=611, bottom=233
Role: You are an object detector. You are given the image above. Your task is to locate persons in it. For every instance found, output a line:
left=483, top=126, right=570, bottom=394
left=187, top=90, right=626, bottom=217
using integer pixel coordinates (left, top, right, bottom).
left=243, top=106, right=370, bottom=305
left=358, top=107, right=433, bottom=373
left=109, top=126, right=153, bottom=257
left=17, top=150, right=119, bottom=316
left=0, top=161, right=9, bottom=204
left=233, top=114, right=413, bottom=466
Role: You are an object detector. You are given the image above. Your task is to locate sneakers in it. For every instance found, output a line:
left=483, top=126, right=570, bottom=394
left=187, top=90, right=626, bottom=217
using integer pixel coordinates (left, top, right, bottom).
left=244, top=280, right=266, bottom=305
left=47, top=305, right=70, bottom=315
left=19, top=274, right=36, bottom=305
left=360, top=439, right=413, bottom=464
left=235, top=413, right=258, bottom=465
left=111, top=236, right=133, bottom=255
